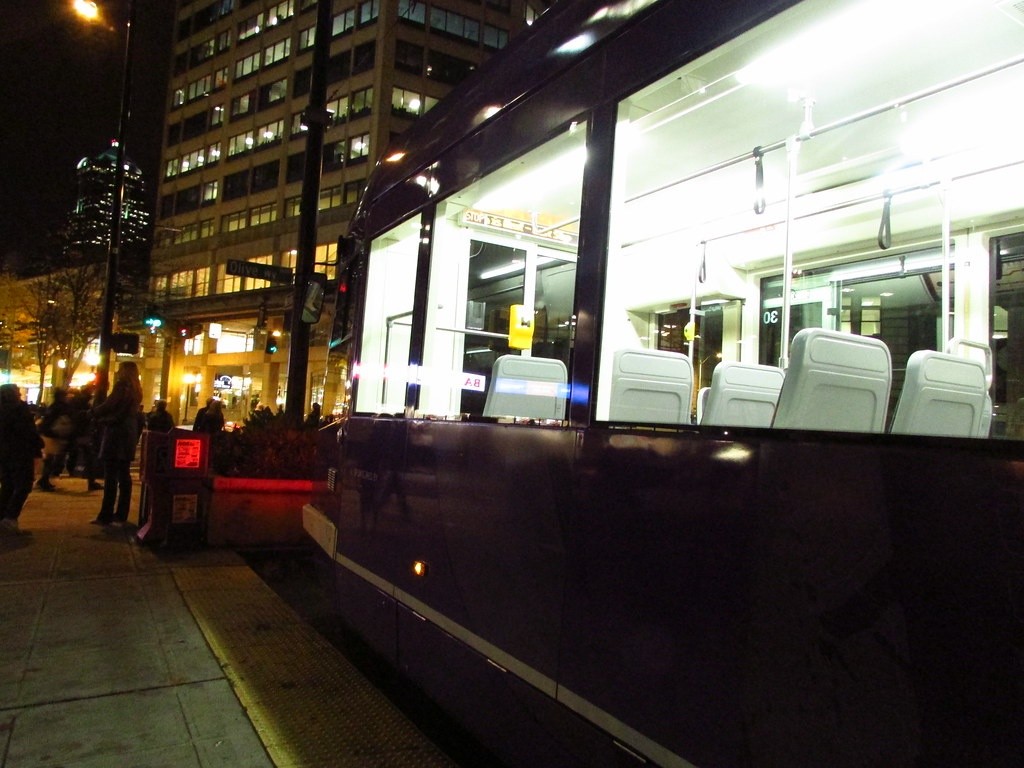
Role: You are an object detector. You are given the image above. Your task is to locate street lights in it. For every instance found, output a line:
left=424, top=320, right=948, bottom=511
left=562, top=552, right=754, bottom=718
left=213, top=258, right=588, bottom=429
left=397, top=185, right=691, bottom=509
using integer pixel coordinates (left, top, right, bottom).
left=71, top=0, right=130, bottom=478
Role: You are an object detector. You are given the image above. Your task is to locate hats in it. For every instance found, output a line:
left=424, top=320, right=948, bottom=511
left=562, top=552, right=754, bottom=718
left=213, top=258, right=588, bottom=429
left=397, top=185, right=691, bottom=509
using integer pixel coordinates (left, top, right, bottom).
left=154, top=400, right=167, bottom=409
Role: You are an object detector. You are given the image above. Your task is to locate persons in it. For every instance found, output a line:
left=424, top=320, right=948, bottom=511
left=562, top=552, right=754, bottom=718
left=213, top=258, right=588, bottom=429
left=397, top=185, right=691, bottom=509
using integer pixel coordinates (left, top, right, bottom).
left=192, top=397, right=226, bottom=431
left=250, top=393, right=321, bottom=425
left=136, top=399, right=174, bottom=444
left=0, top=384, right=43, bottom=535
left=29, top=360, right=143, bottom=526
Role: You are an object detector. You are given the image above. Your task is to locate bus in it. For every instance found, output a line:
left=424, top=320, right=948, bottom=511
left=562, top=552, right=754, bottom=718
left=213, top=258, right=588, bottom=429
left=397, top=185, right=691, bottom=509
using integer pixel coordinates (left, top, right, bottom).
left=302, top=0, right=1024, bottom=767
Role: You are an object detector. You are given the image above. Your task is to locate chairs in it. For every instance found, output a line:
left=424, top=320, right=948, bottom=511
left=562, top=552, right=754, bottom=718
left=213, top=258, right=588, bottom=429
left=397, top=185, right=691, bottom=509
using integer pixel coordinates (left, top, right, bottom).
left=483, top=326, right=995, bottom=439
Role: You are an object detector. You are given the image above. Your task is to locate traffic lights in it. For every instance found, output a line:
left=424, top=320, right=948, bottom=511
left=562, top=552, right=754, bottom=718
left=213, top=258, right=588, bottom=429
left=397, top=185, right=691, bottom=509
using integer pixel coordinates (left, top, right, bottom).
left=175, top=324, right=199, bottom=340
left=144, top=313, right=166, bottom=330
left=264, top=330, right=277, bottom=355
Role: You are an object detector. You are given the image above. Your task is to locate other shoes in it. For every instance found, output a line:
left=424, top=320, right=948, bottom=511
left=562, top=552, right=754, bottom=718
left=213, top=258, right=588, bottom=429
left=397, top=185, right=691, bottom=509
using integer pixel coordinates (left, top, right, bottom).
left=35, top=479, right=56, bottom=491
left=88, top=482, right=104, bottom=490
left=0, top=519, right=32, bottom=536
left=90, top=519, right=113, bottom=526
left=113, top=513, right=126, bottom=522
